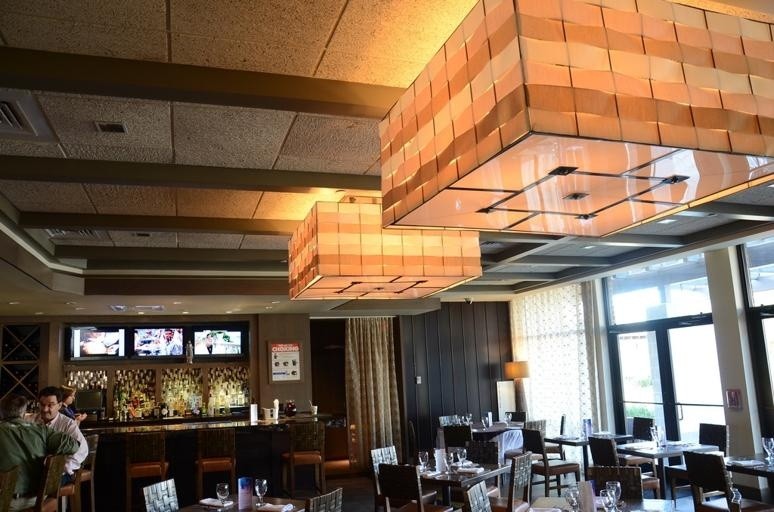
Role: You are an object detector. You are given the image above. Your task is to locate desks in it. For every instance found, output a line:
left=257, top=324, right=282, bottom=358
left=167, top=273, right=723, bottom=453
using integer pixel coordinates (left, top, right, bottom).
left=617, top=440, right=719, bottom=500
left=543, top=432, right=634, bottom=482
left=723, top=455, right=773, bottom=511
left=530, top=496, right=675, bottom=511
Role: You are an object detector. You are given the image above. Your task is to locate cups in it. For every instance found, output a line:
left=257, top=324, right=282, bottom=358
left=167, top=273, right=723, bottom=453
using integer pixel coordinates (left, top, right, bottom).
left=272, top=409, right=279, bottom=420
left=310, top=406, right=318, bottom=416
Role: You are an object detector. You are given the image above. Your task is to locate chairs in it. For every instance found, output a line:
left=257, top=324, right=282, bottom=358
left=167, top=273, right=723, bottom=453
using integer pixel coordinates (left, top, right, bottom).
left=587, top=465, right=643, bottom=500
left=544, top=415, right=568, bottom=479
left=0, top=421, right=342, bottom=512
left=370, top=410, right=546, bottom=511
left=587, top=435, right=660, bottom=499
left=520, top=427, right=581, bottom=497
left=667, top=423, right=732, bottom=508
left=682, top=450, right=768, bottom=512
left=616, top=416, right=657, bottom=478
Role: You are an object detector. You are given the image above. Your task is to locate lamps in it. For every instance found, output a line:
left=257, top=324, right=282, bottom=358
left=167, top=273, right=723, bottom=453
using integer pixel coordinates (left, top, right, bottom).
left=377, top=0, right=774, bottom=239
left=287, top=194, right=483, bottom=301
left=503, top=360, right=530, bottom=412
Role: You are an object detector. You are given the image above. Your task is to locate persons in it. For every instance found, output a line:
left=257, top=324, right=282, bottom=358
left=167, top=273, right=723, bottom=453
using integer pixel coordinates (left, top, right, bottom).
left=60, top=388, right=87, bottom=426
left=205, top=333, right=215, bottom=354
left=0, top=390, right=79, bottom=512
left=25, top=386, right=89, bottom=489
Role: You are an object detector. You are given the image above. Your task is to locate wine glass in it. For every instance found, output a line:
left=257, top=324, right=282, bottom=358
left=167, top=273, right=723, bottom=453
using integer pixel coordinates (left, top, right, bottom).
left=760, top=437, right=774, bottom=467
left=564, top=481, right=625, bottom=512
left=649, top=426, right=667, bottom=451
left=255, top=478, right=269, bottom=510
left=447, top=412, right=513, bottom=431
left=418, top=448, right=474, bottom=475
left=215, top=483, right=230, bottom=511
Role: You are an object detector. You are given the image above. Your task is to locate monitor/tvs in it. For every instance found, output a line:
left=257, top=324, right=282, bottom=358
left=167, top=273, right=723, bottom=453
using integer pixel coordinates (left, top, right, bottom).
left=74, top=390, right=103, bottom=410
left=189, top=321, right=248, bottom=363
left=64, top=323, right=129, bottom=362
left=129, top=326, right=187, bottom=360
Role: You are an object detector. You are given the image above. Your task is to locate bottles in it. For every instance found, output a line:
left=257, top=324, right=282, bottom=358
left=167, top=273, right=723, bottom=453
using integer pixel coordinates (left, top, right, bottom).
left=112, top=392, right=233, bottom=423
left=185, top=341, right=193, bottom=365
